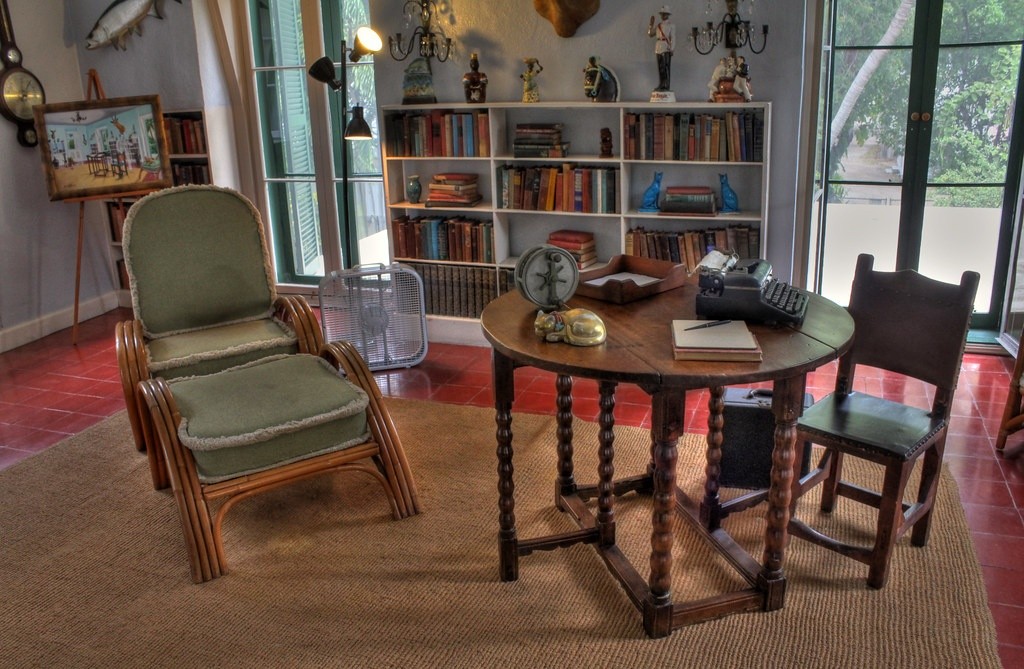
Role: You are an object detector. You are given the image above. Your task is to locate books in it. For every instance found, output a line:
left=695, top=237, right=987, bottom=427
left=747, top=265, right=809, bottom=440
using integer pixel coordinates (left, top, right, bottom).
left=110, top=117, right=210, bottom=290
left=384, top=107, right=764, bottom=318
left=671, top=324, right=763, bottom=362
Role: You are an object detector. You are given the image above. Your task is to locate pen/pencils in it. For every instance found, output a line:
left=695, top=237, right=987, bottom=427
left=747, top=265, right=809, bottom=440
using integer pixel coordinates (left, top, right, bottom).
left=684, top=319, right=731, bottom=331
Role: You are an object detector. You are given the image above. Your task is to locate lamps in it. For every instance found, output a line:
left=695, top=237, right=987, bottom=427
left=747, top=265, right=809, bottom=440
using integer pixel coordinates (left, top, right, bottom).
left=307, top=26, right=382, bottom=349
left=685, top=0, right=771, bottom=60
left=386, top=0, right=453, bottom=73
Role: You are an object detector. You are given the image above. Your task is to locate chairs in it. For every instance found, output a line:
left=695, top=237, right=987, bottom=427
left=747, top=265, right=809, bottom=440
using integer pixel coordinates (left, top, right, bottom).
left=789, top=254, right=981, bottom=591
left=114, top=179, right=324, bottom=450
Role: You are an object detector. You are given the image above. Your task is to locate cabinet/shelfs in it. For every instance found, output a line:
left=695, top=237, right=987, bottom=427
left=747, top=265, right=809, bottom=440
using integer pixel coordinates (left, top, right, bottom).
left=103, top=106, right=211, bottom=290
left=378, top=102, right=773, bottom=349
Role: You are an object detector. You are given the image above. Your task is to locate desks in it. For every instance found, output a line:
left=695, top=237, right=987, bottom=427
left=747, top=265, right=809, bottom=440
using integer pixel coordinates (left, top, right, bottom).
left=479, top=269, right=856, bottom=638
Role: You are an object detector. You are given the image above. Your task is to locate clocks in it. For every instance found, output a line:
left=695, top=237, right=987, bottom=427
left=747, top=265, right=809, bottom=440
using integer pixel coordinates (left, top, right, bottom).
left=0, top=0, right=45, bottom=147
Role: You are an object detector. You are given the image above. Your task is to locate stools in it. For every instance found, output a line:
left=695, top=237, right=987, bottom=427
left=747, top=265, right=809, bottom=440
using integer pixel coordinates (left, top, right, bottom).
left=137, top=341, right=423, bottom=586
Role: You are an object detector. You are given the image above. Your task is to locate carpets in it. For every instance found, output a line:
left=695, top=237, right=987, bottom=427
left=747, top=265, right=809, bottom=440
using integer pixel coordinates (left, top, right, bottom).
left=0, top=392, right=1006, bottom=669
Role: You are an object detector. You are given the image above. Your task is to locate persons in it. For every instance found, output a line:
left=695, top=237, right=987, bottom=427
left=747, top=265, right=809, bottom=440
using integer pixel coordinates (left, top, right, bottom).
left=706, top=56, right=752, bottom=103
left=648, top=5, right=676, bottom=92
left=519, top=57, right=543, bottom=103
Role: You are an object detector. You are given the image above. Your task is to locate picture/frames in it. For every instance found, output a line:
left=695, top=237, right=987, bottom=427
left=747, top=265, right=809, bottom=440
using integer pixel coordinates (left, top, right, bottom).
left=31, top=94, right=175, bottom=202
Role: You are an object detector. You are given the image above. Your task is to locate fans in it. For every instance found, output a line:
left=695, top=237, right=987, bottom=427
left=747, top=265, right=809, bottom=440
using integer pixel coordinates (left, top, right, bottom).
left=319, top=263, right=429, bottom=372
